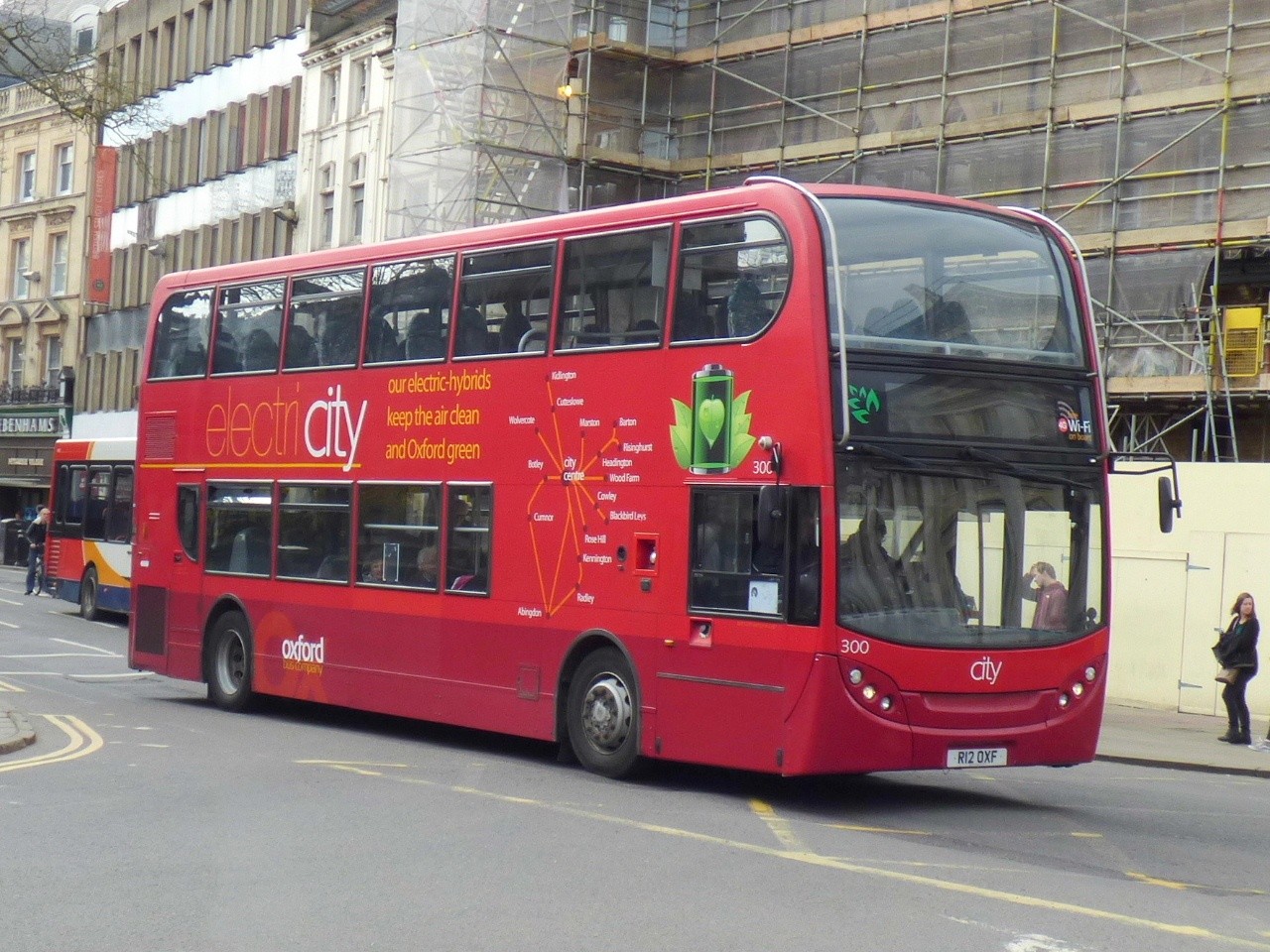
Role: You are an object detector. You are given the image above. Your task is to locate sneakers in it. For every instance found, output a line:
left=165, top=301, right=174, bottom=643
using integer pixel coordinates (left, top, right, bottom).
left=1249, top=739, right=1269, bottom=752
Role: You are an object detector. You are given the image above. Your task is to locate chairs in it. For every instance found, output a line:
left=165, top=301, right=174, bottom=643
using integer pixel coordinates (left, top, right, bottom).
left=230, top=525, right=489, bottom=594
left=175, top=271, right=979, bottom=379
left=694, top=522, right=740, bottom=602
left=66, top=496, right=134, bottom=542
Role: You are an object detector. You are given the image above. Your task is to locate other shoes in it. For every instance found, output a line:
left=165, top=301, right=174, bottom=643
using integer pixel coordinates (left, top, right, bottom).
left=1230, top=727, right=1252, bottom=744
left=1218, top=724, right=1239, bottom=741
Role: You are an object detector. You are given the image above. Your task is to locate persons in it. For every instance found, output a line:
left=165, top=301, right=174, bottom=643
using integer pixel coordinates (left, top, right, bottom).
left=1021, top=562, right=1068, bottom=629
left=839, top=510, right=899, bottom=607
left=1220, top=593, right=1270, bottom=753
left=13, top=504, right=49, bottom=595
left=363, top=547, right=454, bottom=588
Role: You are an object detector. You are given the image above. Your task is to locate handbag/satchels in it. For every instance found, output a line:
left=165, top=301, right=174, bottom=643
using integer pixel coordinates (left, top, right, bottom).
left=1215, top=666, right=1240, bottom=686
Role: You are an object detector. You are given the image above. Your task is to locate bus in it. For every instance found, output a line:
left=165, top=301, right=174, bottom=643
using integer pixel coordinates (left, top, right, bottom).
left=41, top=440, right=138, bottom=622
left=127, top=175, right=1182, bottom=780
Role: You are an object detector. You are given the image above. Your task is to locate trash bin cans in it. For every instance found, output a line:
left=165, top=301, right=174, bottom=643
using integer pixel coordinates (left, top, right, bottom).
left=0, top=518, right=31, bottom=566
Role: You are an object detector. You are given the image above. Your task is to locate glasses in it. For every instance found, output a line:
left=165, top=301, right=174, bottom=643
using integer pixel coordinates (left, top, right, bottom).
left=879, top=536, right=887, bottom=542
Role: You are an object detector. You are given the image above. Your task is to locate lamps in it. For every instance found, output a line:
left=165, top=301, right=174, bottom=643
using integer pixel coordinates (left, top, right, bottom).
left=21, top=270, right=40, bottom=282
left=148, top=244, right=167, bottom=258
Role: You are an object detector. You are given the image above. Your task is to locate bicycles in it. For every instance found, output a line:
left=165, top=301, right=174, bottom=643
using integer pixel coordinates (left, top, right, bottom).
left=32, top=544, right=44, bottom=596
